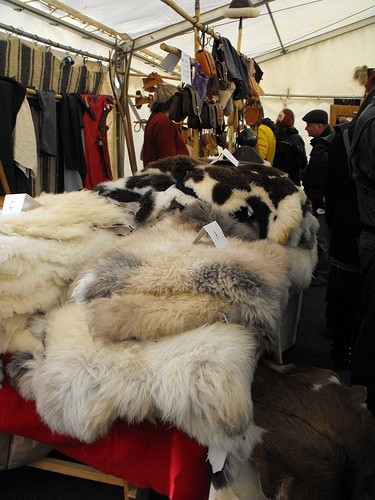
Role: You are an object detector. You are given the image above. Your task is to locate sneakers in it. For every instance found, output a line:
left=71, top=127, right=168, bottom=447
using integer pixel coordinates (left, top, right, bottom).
left=309, top=274, right=328, bottom=287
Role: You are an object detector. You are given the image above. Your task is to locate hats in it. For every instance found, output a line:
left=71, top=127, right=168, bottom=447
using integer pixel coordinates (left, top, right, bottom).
left=302, top=110, right=328, bottom=124
left=153, top=84, right=179, bottom=103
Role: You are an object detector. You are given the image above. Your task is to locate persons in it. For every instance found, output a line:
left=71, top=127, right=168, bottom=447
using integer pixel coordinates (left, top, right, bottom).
left=302, top=109, right=338, bottom=208
left=243, top=100, right=276, bottom=167
left=272, top=108, right=308, bottom=177
left=350, top=93, right=375, bottom=291
left=141, top=84, right=190, bottom=173
